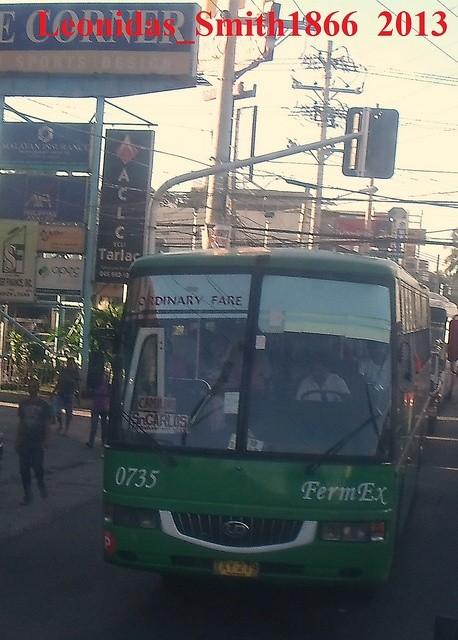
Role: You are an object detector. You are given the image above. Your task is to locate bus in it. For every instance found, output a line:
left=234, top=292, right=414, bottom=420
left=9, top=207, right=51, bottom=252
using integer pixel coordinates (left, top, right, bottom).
left=102, top=248, right=432, bottom=587
left=430, top=291, right=458, bottom=408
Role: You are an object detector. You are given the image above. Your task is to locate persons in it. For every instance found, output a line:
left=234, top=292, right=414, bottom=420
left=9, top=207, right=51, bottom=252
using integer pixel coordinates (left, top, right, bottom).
left=296, top=352, right=354, bottom=404
left=135, top=347, right=185, bottom=391
left=86, top=374, right=111, bottom=448
left=51, top=357, right=81, bottom=437
left=15, top=378, right=52, bottom=506
